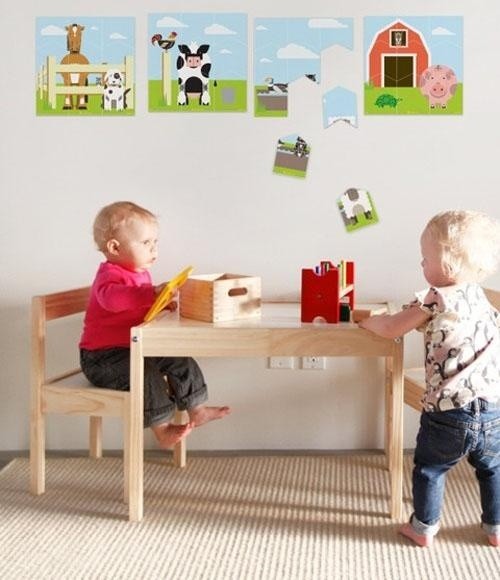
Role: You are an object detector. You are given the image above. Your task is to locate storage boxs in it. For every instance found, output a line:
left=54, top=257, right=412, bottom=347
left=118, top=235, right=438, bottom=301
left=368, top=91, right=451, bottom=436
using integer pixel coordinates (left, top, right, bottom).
left=180, top=271, right=260, bottom=323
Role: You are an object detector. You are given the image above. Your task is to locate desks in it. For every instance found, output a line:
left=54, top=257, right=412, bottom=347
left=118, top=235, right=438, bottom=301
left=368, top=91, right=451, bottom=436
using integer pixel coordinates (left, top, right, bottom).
left=128, top=300, right=406, bottom=526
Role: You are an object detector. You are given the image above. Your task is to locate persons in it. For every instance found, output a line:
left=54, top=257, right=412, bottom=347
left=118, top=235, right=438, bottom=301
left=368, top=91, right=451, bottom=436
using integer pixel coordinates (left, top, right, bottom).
left=355, top=211, right=500, bottom=551
left=78, top=201, right=230, bottom=450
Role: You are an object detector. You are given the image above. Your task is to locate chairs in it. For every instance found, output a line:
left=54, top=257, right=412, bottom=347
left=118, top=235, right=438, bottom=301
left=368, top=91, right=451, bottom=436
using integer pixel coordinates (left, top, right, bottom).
left=28, top=284, right=188, bottom=496
left=404, top=285, right=500, bottom=414
